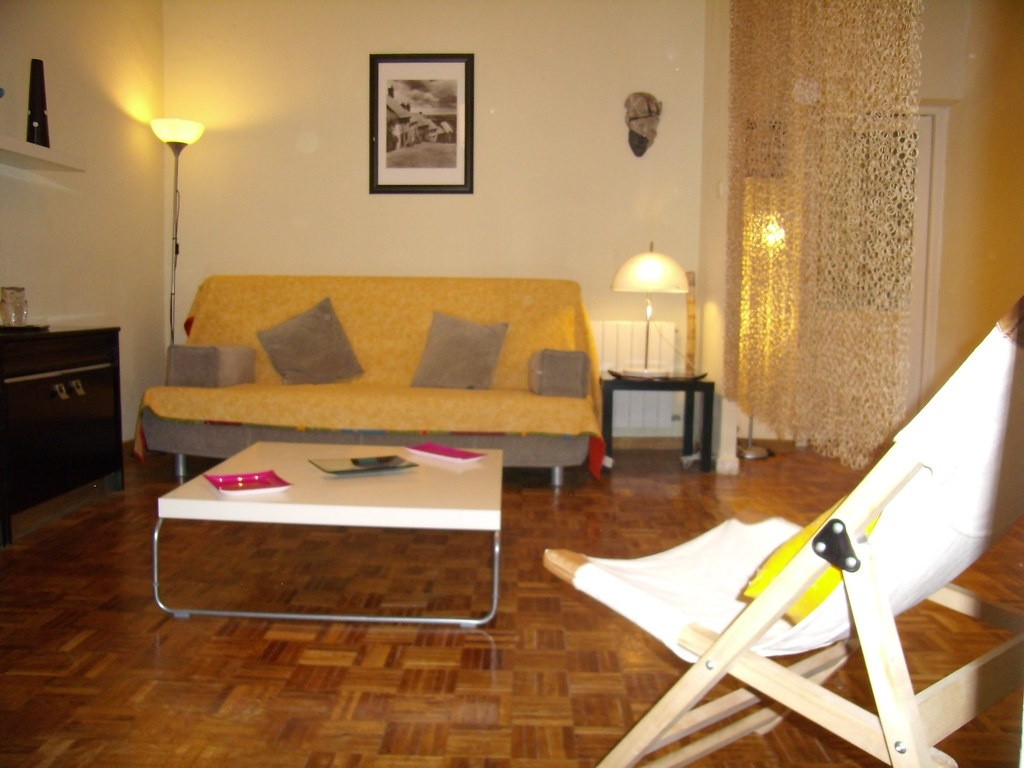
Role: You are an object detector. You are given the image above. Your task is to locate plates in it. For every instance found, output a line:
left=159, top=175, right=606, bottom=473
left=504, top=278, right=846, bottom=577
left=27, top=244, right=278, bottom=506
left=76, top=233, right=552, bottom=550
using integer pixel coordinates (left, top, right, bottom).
left=402, top=442, right=488, bottom=465
left=309, top=455, right=418, bottom=477
left=202, top=470, right=293, bottom=497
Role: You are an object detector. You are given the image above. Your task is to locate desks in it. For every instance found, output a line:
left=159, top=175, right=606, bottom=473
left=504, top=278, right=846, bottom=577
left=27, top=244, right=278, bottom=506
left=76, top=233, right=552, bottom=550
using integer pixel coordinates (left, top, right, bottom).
left=602, top=375, right=716, bottom=471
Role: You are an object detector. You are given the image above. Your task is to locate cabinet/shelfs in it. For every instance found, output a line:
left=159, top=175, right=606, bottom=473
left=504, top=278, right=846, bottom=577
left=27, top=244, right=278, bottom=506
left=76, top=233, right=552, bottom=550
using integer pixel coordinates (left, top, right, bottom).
left=0, top=325, right=125, bottom=547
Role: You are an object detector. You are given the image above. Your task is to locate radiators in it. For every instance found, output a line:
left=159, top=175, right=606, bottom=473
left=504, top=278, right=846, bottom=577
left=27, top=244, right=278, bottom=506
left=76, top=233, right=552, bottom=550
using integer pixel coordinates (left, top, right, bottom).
left=591, top=321, right=683, bottom=430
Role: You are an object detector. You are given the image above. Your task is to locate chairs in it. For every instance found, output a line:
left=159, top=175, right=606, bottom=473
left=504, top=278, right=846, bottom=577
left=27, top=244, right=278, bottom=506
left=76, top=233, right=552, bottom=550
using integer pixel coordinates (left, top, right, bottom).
left=542, top=295, right=1024, bottom=768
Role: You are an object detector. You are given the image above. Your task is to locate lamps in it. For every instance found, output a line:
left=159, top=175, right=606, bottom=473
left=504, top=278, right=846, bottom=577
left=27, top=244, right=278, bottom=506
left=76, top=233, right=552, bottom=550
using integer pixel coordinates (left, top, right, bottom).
left=150, top=118, right=206, bottom=343
left=612, top=242, right=690, bottom=378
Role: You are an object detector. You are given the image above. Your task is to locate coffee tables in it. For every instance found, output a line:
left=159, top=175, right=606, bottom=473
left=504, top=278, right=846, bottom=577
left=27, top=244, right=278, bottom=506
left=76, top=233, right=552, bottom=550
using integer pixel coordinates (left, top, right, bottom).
left=154, top=440, right=505, bottom=627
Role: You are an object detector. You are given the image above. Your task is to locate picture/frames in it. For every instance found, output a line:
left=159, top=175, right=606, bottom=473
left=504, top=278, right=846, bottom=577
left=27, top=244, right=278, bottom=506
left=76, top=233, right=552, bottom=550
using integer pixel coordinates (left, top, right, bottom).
left=370, top=52, right=475, bottom=194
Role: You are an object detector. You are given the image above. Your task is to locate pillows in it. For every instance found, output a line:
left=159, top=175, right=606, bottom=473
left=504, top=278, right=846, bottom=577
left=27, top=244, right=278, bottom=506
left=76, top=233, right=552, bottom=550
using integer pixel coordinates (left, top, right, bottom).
left=528, top=350, right=589, bottom=398
left=257, top=297, right=365, bottom=387
left=415, top=311, right=507, bottom=392
left=168, top=346, right=257, bottom=387
left=745, top=492, right=889, bottom=625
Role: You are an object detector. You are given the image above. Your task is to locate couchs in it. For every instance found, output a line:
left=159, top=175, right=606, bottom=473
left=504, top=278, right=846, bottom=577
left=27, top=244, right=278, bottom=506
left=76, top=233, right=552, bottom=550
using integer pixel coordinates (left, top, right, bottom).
left=133, top=273, right=607, bottom=490
